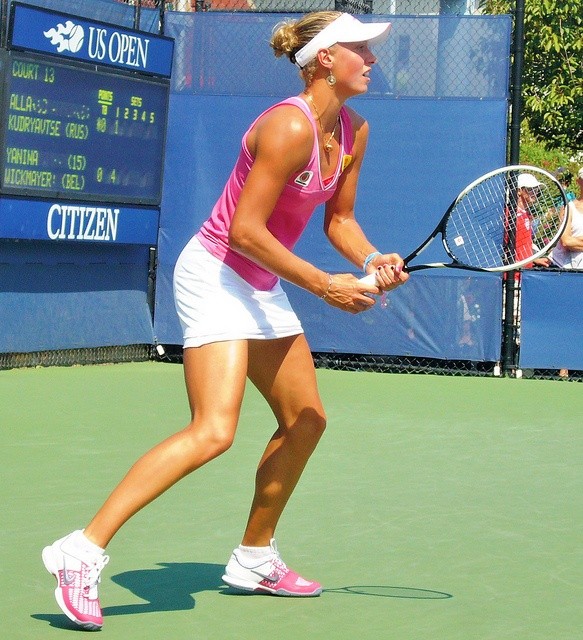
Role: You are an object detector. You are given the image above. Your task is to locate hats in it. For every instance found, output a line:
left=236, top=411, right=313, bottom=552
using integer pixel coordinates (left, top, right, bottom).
left=578, top=167, right=583, bottom=179
left=517, top=174, right=545, bottom=189
left=290, top=12, right=392, bottom=70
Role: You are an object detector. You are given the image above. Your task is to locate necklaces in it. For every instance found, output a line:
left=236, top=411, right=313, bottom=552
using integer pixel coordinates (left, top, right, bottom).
left=306, top=94, right=339, bottom=153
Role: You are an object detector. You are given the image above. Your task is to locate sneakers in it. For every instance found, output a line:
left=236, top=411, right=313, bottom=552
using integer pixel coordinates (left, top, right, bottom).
left=42, top=528, right=110, bottom=631
left=221, top=538, right=323, bottom=597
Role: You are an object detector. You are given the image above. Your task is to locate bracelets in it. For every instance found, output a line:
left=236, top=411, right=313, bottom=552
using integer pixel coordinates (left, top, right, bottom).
left=363, top=251, right=382, bottom=273
left=320, top=273, right=334, bottom=299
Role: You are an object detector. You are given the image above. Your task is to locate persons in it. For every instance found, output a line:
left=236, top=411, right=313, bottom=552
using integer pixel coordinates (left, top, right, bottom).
left=559, top=167, right=583, bottom=380
left=40, top=10, right=411, bottom=630
left=501, top=173, right=552, bottom=378
